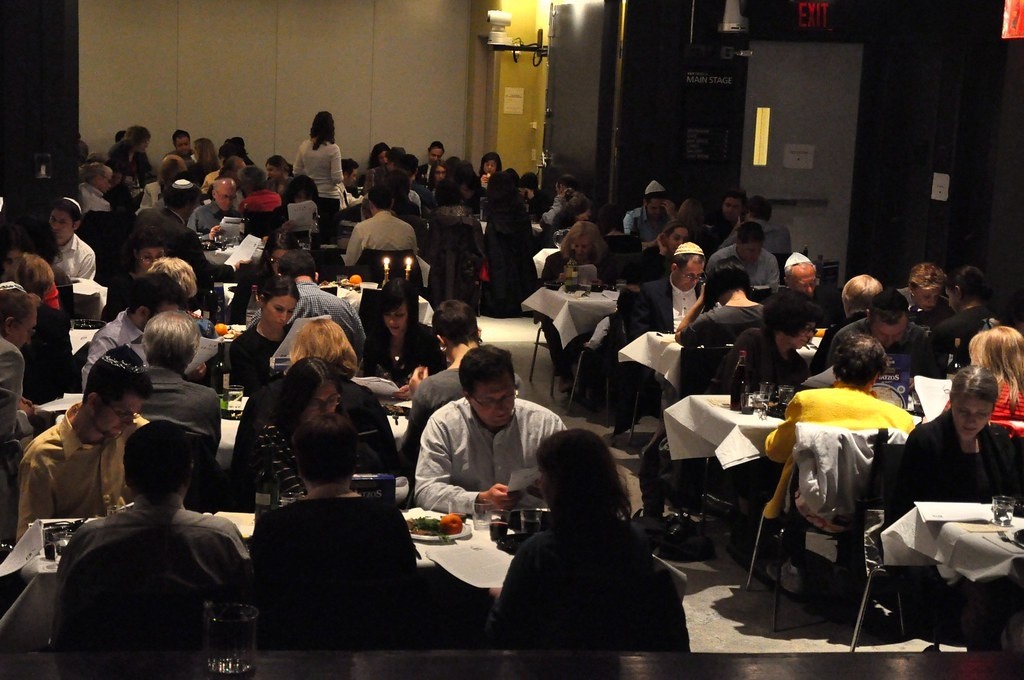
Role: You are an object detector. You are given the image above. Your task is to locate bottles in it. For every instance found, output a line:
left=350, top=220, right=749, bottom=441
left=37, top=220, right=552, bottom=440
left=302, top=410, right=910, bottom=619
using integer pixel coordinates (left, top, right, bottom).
left=254, top=446, right=279, bottom=530
left=946, top=338, right=964, bottom=381
left=564, top=249, right=578, bottom=294
left=730, top=350, right=749, bottom=410
left=211, top=342, right=231, bottom=419
left=309, top=212, right=320, bottom=249
left=245, top=285, right=261, bottom=329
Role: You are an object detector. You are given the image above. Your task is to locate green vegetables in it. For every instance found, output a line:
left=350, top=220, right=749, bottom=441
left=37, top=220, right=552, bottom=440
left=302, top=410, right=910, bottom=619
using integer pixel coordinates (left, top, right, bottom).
left=410, top=517, right=446, bottom=535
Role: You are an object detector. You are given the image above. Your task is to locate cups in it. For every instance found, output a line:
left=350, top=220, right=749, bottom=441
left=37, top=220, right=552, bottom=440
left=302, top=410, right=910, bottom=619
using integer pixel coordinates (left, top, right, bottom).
left=336, top=275, right=347, bottom=283
left=281, top=492, right=303, bottom=504
left=579, top=275, right=603, bottom=296
left=992, top=495, right=1016, bottom=527
left=203, top=603, right=258, bottom=673
left=553, top=227, right=573, bottom=251
left=520, top=509, right=542, bottom=533
left=359, top=282, right=378, bottom=297
left=196, top=232, right=243, bottom=251
left=488, top=509, right=510, bottom=541
left=740, top=382, right=794, bottom=414
left=227, top=385, right=244, bottom=420
left=616, top=279, right=626, bottom=295
left=473, top=500, right=492, bottom=530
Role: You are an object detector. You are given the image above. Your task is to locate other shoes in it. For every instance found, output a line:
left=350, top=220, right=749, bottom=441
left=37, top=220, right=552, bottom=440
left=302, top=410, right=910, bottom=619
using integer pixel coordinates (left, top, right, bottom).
left=670, top=481, right=703, bottom=513
left=577, top=391, right=603, bottom=414
left=917, top=612, right=964, bottom=641
left=779, top=556, right=802, bottom=576
left=704, top=485, right=739, bottom=508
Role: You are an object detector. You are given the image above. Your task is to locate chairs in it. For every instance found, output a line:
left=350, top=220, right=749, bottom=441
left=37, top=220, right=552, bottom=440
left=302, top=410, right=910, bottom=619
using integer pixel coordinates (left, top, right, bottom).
left=57, top=192, right=955, bottom=648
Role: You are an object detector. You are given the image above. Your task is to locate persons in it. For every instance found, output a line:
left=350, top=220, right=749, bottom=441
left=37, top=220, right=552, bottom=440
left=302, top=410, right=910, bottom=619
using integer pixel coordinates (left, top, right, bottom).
left=0, top=275, right=557, bottom=545
left=485, top=428, right=689, bottom=652
left=78, top=111, right=591, bottom=319
left=0, top=196, right=96, bottom=316
left=49, top=419, right=256, bottom=652
left=534, top=178, right=1024, bottom=651
left=251, top=412, right=433, bottom=650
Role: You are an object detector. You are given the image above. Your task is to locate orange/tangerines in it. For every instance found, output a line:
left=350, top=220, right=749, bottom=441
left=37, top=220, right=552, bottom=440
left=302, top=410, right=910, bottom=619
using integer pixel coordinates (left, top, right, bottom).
left=439, top=514, right=462, bottom=535
left=350, top=274, right=362, bottom=284
left=214, top=323, right=228, bottom=336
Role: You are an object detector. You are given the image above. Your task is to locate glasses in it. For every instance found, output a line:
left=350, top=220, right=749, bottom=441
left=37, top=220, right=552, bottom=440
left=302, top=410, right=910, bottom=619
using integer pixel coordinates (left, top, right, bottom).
left=677, top=266, right=708, bottom=284
left=137, top=253, right=166, bottom=265
left=792, top=279, right=821, bottom=289
left=304, top=394, right=343, bottom=410
left=915, top=274, right=949, bottom=285
left=107, top=401, right=138, bottom=425
left=802, top=323, right=819, bottom=338
left=469, top=387, right=519, bottom=409
left=18, top=318, right=36, bottom=340
left=267, top=256, right=280, bottom=264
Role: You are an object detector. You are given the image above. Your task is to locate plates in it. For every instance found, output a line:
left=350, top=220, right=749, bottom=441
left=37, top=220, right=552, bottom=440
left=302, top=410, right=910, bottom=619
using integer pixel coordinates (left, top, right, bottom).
left=404, top=514, right=471, bottom=540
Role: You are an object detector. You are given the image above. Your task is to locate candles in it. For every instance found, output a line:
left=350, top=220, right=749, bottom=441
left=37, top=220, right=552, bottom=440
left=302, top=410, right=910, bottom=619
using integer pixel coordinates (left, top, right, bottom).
left=405, top=257, right=412, bottom=270
left=383, top=257, right=390, bottom=269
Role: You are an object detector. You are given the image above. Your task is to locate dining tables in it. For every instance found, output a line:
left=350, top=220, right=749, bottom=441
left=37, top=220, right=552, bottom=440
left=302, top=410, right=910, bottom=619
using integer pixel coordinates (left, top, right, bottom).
left=618, top=331, right=684, bottom=421
left=663, top=393, right=785, bottom=538
left=67, top=325, right=248, bottom=355
left=69, top=277, right=108, bottom=319
left=521, top=286, right=620, bottom=388
left=19, top=512, right=560, bottom=647
left=879, top=502, right=1024, bottom=652
left=203, top=242, right=339, bottom=266
left=222, top=280, right=434, bottom=327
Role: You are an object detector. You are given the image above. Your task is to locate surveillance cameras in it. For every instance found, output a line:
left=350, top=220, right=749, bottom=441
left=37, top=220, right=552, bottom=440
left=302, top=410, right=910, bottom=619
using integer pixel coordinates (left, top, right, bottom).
left=486, top=11, right=512, bottom=26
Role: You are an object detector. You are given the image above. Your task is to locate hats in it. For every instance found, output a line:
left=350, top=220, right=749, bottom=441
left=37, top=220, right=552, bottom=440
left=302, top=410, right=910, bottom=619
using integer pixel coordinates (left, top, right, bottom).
left=872, top=287, right=909, bottom=312
left=673, top=241, right=705, bottom=260
left=172, top=177, right=194, bottom=190
left=63, top=197, right=84, bottom=216
left=644, top=180, right=666, bottom=195
left=784, top=251, right=815, bottom=274
